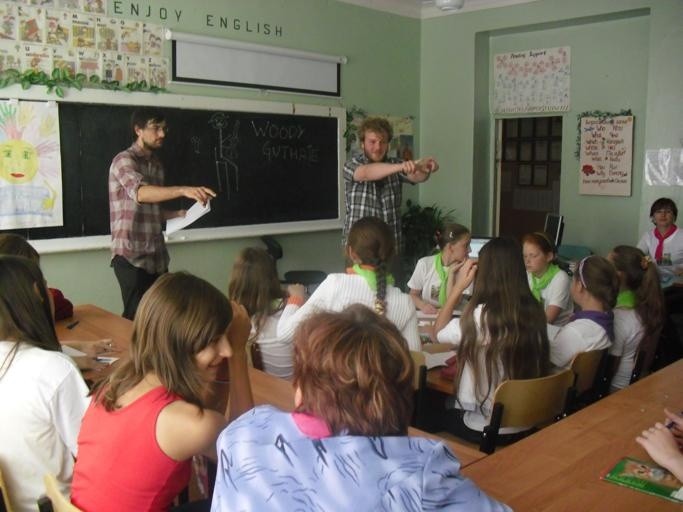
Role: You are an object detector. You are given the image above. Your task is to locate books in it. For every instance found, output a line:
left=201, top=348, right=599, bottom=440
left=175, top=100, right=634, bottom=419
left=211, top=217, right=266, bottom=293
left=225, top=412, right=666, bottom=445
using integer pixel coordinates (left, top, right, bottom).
left=597, top=454, right=682, bottom=504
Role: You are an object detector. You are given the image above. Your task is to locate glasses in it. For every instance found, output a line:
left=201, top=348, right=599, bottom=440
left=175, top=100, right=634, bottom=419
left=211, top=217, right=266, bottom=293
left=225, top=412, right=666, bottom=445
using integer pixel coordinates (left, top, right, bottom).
left=143, top=124, right=169, bottom=133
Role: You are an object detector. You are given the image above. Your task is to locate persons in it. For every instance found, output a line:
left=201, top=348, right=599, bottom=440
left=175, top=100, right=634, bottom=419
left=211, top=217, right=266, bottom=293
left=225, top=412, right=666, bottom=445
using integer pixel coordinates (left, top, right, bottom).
left=635, top=197, right=682, bottom=341
left=605, top=243, right=668, bottom=396
left=342, top=116, right=440, bottom=258
left=522, top=229, right=576, bottom=321
left=428, top=236, right=548, bottom=447
left=0, top=234, right=89, bottom=511
left=105, top=106, right=220, bottom=316
left=633, top=405, right=682, bottom=501
left=0, top=0, right=165, bottom=88
left=72, top=272, right=252, bottom=511
left=546, top=254, right=620, bottom=375
left=209, top=304, right=512, bottom=510
left=232, top=217, right=470, bottom=384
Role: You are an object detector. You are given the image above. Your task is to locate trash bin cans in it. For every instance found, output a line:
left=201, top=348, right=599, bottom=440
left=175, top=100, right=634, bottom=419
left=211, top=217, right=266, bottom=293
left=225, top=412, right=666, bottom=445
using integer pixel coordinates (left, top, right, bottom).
left=284, top=270, right=327, bottom=296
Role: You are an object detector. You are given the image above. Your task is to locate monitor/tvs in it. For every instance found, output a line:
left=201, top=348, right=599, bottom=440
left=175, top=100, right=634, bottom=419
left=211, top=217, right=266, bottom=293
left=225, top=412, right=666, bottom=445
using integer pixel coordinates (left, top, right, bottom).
left=544, top=213, right=564, bottom=248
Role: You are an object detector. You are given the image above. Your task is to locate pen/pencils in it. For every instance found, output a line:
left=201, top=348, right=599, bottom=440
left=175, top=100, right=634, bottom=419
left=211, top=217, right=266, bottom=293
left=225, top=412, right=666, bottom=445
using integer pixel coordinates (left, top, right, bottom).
left=665, top=409, right=683, bottom=429
left=66, top=321, right=81, bottom=329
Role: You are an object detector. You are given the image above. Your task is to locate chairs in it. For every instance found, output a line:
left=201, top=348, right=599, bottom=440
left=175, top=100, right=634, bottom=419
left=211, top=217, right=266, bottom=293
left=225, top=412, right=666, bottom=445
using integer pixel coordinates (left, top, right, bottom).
left=37, top=471, right=80, bottom=512
left=0, top=468, right=12, bottom=512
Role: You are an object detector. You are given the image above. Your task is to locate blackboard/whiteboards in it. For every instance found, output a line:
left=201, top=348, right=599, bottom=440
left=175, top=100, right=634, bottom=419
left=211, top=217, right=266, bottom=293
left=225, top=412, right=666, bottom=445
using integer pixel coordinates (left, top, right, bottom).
left=0, top=90, right=346, bottom=255
